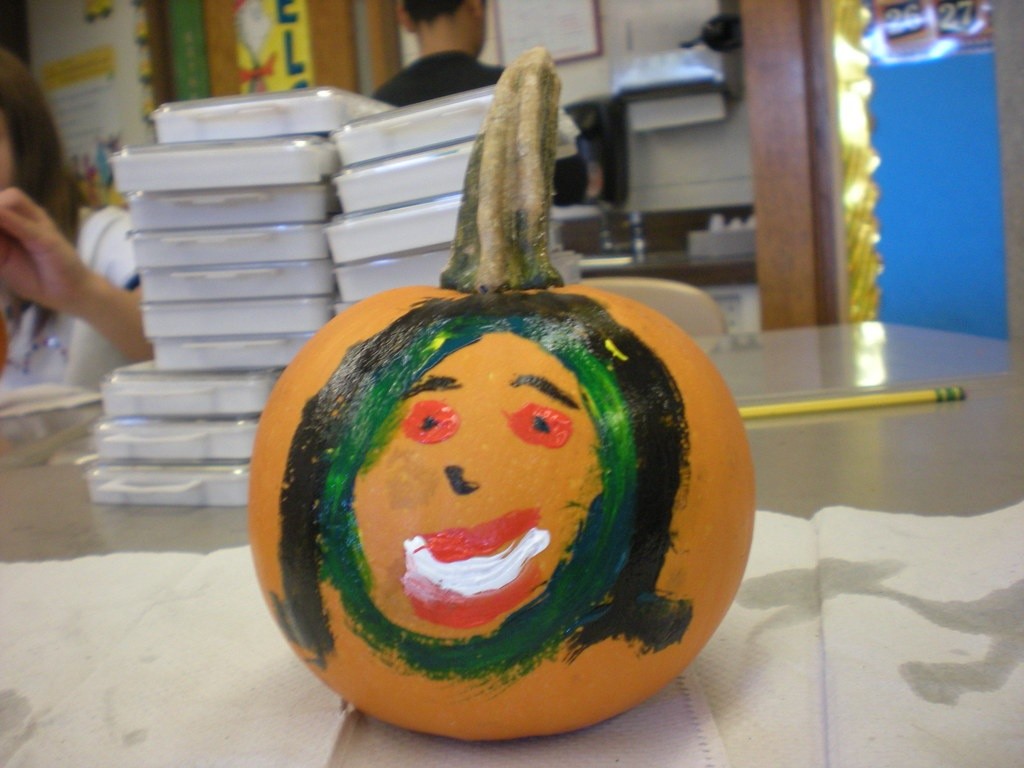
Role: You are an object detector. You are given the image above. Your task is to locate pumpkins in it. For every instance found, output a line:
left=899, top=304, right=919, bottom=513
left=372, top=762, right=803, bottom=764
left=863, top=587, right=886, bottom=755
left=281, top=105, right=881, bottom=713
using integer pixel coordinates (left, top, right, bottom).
left=249, top=48, right=754, bottom=742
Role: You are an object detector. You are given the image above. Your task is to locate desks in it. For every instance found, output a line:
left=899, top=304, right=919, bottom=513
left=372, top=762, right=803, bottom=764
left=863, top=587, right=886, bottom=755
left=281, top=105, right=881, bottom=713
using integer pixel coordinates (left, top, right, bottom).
left=1, top=320, right=1024, bottom=768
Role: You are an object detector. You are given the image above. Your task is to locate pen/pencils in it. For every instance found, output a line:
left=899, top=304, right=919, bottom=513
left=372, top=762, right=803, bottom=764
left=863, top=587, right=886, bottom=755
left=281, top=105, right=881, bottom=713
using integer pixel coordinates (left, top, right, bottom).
left=735, top=383, right=967, bottom=422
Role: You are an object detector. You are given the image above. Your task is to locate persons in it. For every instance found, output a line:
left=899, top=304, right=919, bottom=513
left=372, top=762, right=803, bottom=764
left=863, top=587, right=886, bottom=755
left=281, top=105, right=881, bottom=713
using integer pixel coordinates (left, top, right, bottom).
left=0, top=49, right=154, bottom=381
left=369, top=0, right=602, bottom=206
